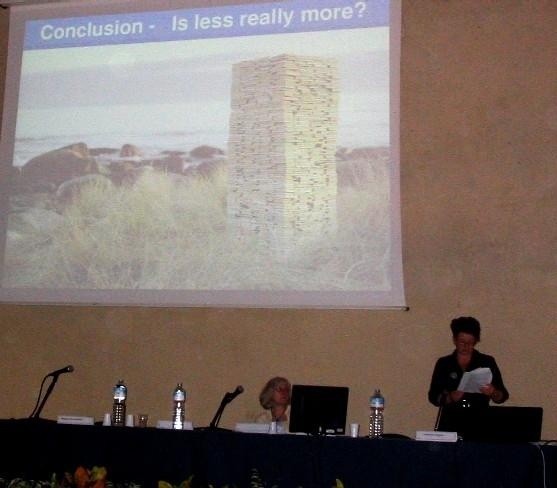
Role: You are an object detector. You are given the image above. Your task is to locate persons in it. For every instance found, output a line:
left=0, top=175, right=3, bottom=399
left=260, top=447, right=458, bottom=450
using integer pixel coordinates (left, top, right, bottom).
left=427, top=317, right=508, bottom=432
left=255, top=375, right=291, bottom=431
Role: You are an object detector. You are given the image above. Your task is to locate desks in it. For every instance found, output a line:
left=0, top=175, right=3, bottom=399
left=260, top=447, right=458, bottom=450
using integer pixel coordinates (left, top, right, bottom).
left=0, top=418, right=557, bottom=488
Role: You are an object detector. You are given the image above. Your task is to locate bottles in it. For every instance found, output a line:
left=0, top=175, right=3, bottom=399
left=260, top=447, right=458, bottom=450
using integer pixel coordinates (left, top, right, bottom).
left=369, top=389, right=383, bottom=437
left=173, top=384, right=185, bottom=429
left=112, top=380, right=127, bottom=425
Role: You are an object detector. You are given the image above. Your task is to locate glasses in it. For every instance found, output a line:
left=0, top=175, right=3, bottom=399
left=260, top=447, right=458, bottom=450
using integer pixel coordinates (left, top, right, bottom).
left=456, top=339, right=477, bottom=347
left=271, top=386, right=290, bottom=393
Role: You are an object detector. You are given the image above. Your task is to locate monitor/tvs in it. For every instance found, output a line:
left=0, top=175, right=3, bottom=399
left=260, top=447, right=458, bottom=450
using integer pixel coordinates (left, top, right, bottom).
left=289, top=385, right=349, bottom=436
left=467, top=407, right=543, bottom=445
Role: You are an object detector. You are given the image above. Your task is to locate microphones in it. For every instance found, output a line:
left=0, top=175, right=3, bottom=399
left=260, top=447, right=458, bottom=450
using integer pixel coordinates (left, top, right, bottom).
left=51, top=365, right=74, bottom=377
left=228, top=386, right=244, bottom=403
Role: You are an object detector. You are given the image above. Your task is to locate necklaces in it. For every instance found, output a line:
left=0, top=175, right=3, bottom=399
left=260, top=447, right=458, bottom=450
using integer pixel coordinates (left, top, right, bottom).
left=273, top=414, right=282, bottom=421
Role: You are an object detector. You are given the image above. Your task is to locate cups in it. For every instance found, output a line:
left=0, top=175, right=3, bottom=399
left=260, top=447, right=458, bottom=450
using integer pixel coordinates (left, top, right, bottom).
left=126, top=415, right=136, bottom=427
left=137, top=414, right=148, bottom=427
left=268, top=422, right=278, bottom=434
left=102, top=413, right=111, bottom=426
left=349, top=424, right=360, bottom=438
